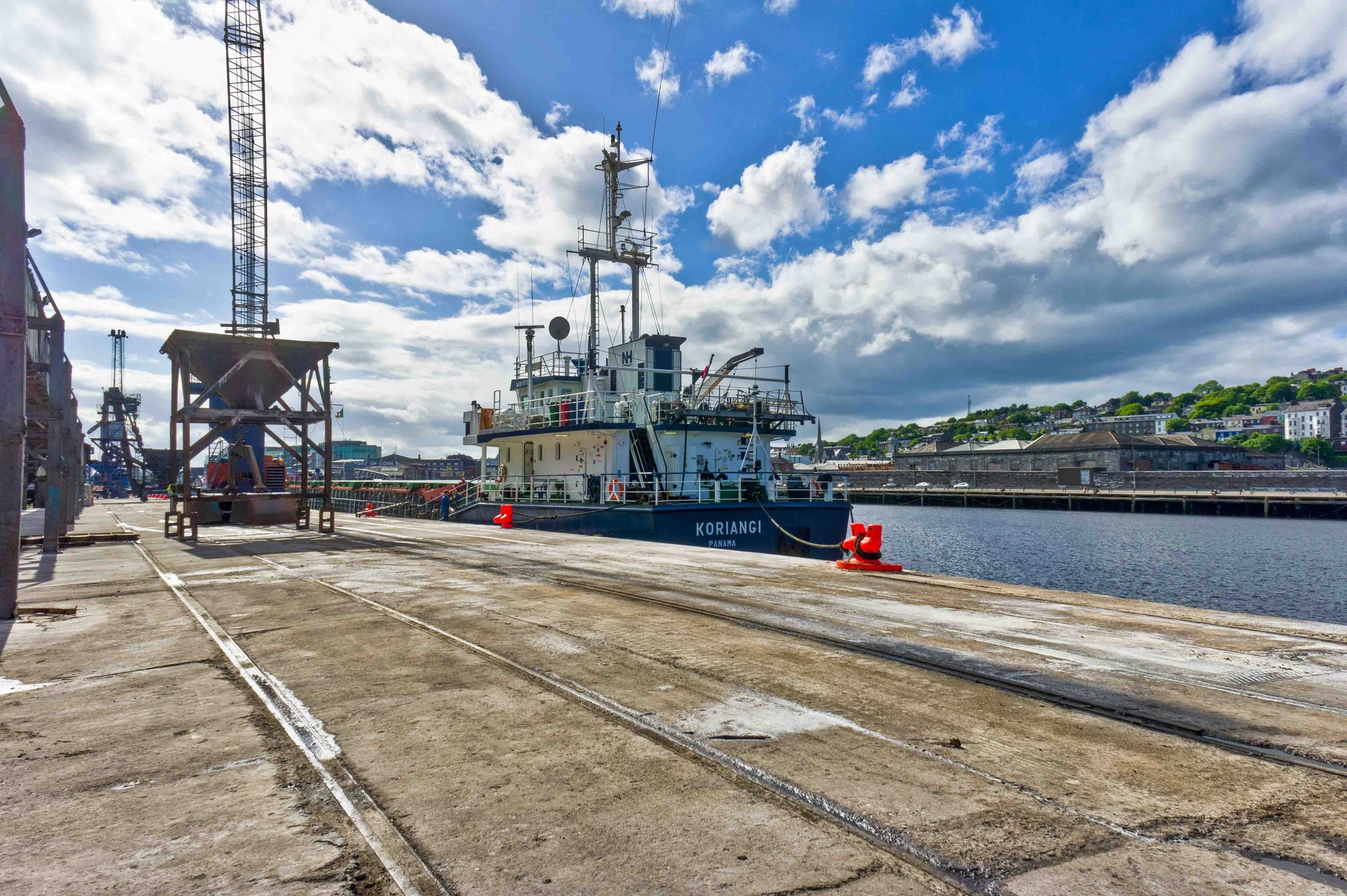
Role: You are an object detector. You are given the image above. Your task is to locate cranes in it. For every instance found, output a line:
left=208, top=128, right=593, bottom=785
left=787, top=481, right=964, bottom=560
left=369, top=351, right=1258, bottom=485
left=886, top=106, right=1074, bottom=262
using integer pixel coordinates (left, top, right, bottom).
left=88, top=329, right=151, bottom=500
left=185, top=1, right=291, bottom=493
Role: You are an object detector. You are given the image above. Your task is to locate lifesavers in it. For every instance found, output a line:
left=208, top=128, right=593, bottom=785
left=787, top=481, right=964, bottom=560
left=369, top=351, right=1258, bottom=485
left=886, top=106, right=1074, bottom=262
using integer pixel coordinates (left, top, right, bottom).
left=808, top=480, right=820, bottom=498
left=499, top=464, right=506, bottom=480
left=608, top=479, right=624, bottom=501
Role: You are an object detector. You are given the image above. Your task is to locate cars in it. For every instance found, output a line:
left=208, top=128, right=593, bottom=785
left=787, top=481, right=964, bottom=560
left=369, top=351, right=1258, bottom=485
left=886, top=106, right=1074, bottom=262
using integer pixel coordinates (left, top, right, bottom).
left=915, top=482, right=932, bottom=488
left=838, top=483, right=852, bottom=488
left=882, top=483, right=897, bottom=488
left=953, top=482, right=969, bottom=488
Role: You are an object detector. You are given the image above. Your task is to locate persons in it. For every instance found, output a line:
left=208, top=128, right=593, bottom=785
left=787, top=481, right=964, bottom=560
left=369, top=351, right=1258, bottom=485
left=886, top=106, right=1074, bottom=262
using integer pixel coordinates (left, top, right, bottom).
left=441, top=490, right=453, bottom=521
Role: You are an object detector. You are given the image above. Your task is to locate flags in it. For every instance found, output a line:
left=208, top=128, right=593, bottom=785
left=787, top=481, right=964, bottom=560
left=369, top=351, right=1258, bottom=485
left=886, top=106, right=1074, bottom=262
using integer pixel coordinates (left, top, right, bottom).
left=335, top=408, right=344, bottom=418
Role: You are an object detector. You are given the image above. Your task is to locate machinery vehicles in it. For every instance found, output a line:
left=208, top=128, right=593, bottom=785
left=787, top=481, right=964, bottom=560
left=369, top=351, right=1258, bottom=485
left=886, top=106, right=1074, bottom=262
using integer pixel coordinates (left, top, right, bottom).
left=86, top=462, right=132, bottom=499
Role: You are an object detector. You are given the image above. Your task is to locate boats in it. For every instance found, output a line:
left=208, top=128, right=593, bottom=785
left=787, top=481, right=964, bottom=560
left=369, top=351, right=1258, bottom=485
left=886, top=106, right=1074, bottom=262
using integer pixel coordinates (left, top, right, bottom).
left=420, top=0, right=855, bottom=561
